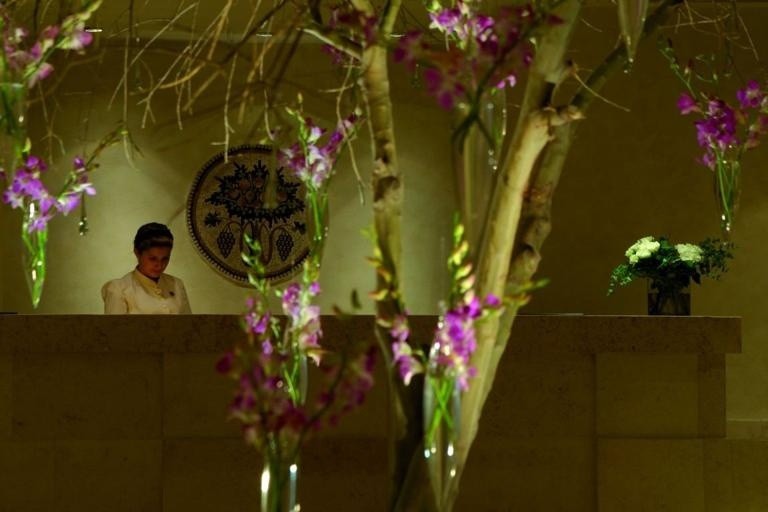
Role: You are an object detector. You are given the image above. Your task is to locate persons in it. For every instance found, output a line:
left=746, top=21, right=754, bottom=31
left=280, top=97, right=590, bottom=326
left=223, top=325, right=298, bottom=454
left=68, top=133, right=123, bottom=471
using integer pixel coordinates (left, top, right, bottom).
left=100, top=221, right=199, bottom=316
